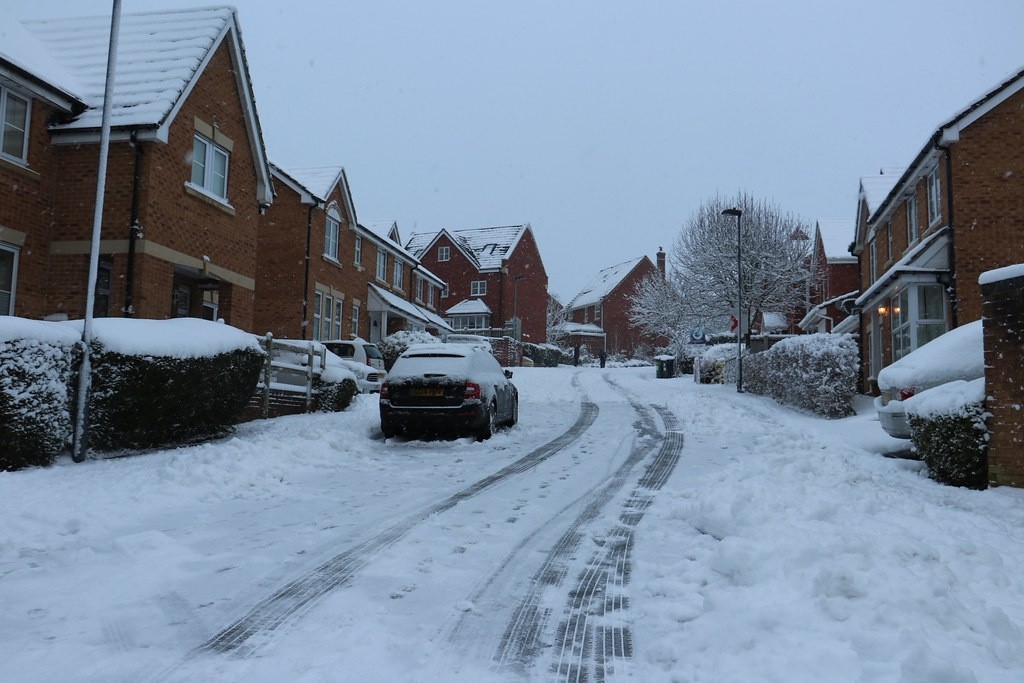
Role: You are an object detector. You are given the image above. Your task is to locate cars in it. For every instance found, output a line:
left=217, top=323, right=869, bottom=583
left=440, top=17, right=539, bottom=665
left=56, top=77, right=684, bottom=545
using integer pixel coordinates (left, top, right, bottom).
left=379, top=341, right=521, bottom=441
left=874, top=318, right=983, bottom=439
left=341, top=357, right=383, bottom=395
left=512, top=274, right=529, bottom=339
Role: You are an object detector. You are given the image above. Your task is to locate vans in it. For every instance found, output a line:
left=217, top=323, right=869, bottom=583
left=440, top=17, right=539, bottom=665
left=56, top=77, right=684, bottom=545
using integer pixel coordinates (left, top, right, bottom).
left=325, top=336, right=386, bottom=368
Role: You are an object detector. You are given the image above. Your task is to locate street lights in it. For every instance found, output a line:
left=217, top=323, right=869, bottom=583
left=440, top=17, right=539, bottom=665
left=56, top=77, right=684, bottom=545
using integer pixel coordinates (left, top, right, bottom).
left=721, top=207, right=744, bottom=392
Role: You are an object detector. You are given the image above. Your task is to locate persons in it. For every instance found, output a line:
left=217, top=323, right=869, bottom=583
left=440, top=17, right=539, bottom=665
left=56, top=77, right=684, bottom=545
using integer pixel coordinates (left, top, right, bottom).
left=598, top=348, right=607, bottom=367
left=573, top=344, right=582, bottom=367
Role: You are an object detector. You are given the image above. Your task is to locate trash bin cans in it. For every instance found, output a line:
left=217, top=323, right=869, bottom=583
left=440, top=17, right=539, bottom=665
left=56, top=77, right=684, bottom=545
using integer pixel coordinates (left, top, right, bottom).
left=654, top=355, right=676, bottom=379
